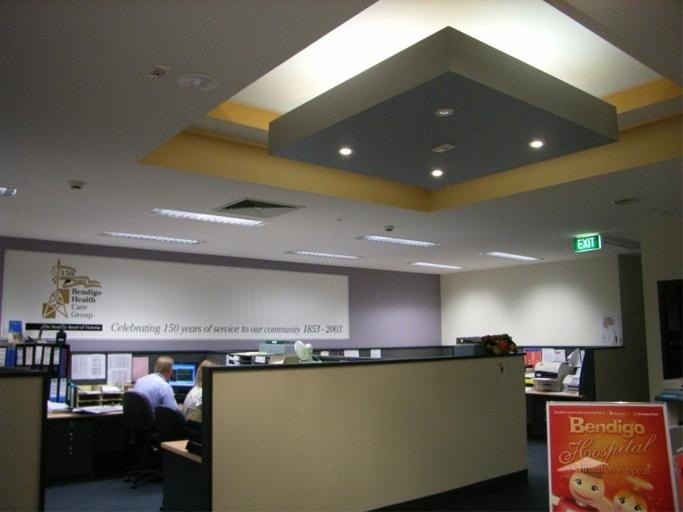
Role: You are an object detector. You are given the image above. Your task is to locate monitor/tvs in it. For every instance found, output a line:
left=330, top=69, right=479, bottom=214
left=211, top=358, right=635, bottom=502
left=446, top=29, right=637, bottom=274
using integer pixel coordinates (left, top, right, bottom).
left=167, top=362, right=197, bottom=388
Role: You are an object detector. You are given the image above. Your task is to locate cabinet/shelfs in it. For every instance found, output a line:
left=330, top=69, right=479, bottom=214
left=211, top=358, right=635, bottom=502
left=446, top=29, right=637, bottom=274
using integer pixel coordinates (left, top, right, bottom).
left=73, top=384, right=123, bottom=409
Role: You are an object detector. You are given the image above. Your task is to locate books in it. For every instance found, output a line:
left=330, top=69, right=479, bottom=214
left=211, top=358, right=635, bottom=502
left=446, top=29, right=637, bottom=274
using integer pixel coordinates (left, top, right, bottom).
left=0, top=343, right=71, bottom=414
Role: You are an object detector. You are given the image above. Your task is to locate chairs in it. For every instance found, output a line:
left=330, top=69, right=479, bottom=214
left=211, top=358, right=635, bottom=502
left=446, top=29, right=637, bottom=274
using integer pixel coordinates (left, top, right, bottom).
left=121, top=391, right=157, bottom=490
left=153, top=404, right=190, bottom=440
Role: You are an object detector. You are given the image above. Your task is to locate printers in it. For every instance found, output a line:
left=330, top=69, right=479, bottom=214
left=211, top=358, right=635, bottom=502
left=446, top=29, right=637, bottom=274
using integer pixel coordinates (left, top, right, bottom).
left=533, top=362, right=570, bottom=391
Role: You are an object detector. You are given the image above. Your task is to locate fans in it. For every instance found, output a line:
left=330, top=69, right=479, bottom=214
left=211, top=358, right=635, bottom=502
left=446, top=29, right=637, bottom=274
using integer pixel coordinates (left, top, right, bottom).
left=294, top=340, right=313, bottom=364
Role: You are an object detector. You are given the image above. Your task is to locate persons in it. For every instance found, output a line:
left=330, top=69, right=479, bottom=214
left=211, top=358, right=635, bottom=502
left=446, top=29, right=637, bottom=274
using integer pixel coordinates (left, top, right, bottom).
left=182, top=360, right=218, bottom=423
left=132, top=354, right=177, bottom=422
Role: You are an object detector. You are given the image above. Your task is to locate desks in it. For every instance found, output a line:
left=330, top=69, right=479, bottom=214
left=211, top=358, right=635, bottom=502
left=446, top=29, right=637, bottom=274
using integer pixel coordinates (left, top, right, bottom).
left=525, top=384, right=594, bottom=441
left=47, top=405, right=123, bottom=481
left=160, top=440, right=211, bottom=512
